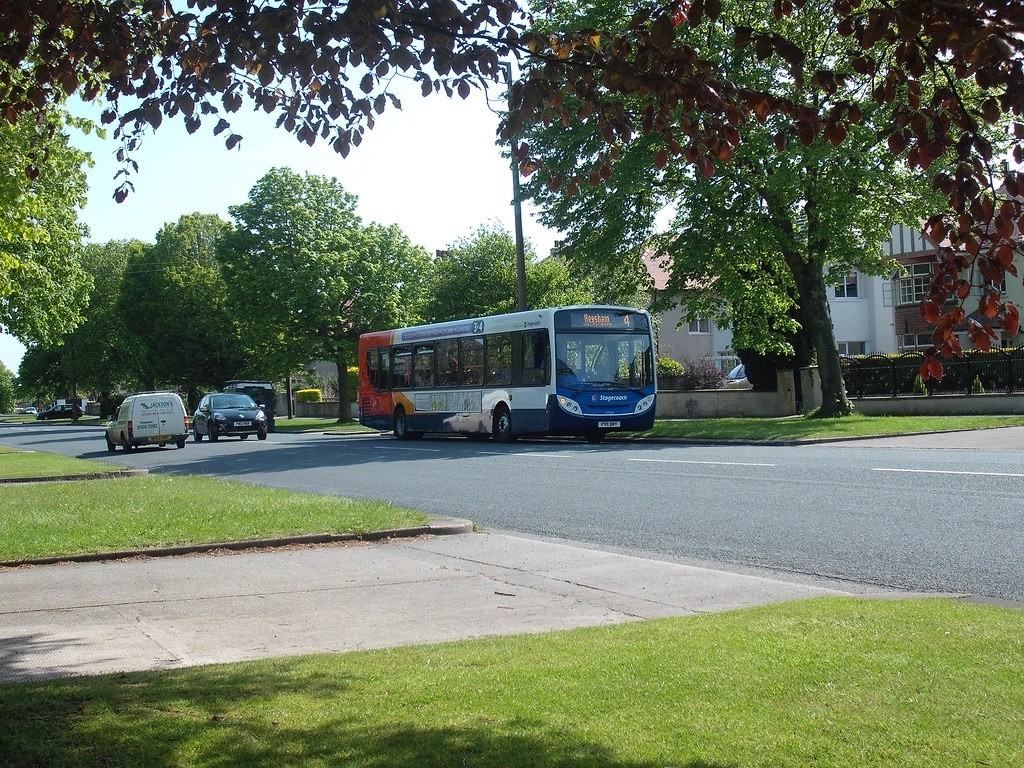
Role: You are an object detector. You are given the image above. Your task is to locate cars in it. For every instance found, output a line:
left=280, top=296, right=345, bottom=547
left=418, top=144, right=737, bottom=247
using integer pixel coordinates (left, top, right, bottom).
left=718, top=362, right=753, bottom=389
left=37, top=404, right=82, bottom=421
left=192, top=393, right=268, bottom=442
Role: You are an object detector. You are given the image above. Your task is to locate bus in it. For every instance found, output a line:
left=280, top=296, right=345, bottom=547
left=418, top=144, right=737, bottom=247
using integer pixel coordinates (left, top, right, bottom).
left=358, top=305, right=658, bottom=445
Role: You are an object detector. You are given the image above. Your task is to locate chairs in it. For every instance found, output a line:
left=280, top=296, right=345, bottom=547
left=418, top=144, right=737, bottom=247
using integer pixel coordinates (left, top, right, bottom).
left=371, top=364, right=613, bottom=385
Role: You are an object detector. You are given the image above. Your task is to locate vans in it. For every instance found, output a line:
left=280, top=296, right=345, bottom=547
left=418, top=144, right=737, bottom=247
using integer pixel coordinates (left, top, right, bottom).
left=105, top=392, right=190, bottom=453
left=224, top=380, right=276, bottom=432
